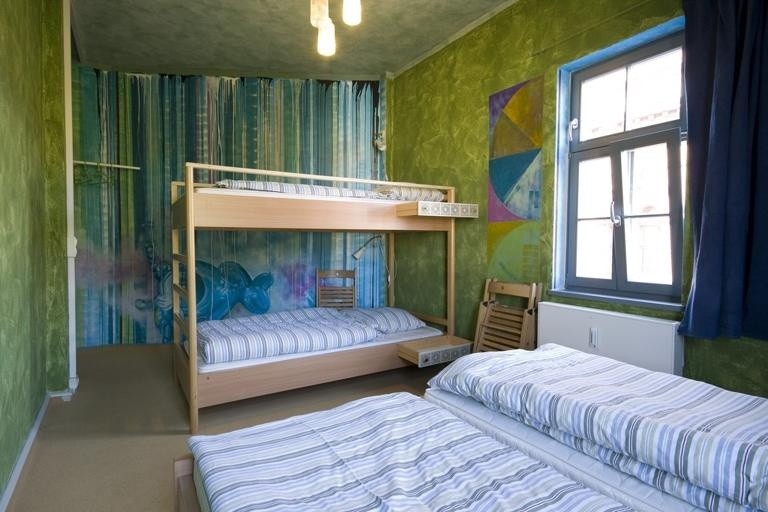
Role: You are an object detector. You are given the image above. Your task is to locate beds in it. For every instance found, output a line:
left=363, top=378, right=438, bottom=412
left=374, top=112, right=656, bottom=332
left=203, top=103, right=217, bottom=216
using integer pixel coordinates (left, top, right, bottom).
left=315, top=267, right=357, bottom=308
left=424, top=343, right=768, bottom=512
left=173, top=392, right=646, bottom=512
left=170, top=161, right=479, bottom=434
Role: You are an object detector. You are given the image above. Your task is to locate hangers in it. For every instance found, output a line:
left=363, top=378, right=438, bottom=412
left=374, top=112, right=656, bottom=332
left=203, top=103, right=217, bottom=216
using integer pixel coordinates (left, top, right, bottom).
left=74, top=160, right=116, bottom=185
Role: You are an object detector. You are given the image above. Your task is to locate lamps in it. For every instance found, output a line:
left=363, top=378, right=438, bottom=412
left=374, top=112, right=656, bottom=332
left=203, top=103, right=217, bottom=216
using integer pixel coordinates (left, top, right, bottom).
left=352, top=236, right=382, bottom=260
left=310, top=0, right=361, bottom=56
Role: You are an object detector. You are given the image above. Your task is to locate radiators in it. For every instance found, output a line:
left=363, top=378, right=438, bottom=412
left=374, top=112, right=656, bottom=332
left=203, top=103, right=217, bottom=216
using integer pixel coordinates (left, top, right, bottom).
left=537, top=301, right=684, bottom=377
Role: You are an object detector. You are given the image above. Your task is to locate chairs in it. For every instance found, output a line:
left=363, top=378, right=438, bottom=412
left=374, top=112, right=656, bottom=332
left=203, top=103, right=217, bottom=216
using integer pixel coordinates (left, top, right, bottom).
left=473, top=279, right=543, bottom=352
